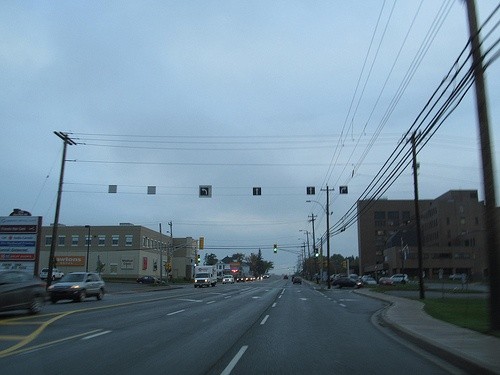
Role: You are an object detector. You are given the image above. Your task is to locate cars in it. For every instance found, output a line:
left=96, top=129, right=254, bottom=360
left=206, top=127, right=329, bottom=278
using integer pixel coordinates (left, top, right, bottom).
left=335, top=277, right=363, bottom=289
left=256, top=275, right=264, bottom=281
left=136, top=275, right=166, bottom=285
left=262, top=274, right=271, bottom=279
left=0, top=270, right=49, bottom=315
left=447, top=272, right=464, bottom=280
left=236, top=275, right=256, bottom=282
left=47, top=271, right=106, bottom=303
left=222, top=274, right=235, bottom=284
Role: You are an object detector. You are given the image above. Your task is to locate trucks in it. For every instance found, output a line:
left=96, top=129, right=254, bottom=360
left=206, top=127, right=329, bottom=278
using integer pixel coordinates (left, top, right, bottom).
left=193, top=265, right=218, bottom=288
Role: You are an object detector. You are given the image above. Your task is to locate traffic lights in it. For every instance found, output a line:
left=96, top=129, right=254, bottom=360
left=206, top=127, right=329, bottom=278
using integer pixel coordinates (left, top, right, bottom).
left=315, top=247, right=319, bottom=262
left=273, top=244, right=278, bottom=254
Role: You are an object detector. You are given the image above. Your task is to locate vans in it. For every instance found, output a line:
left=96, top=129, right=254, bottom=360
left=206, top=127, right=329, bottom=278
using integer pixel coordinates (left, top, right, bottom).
left=39, top=268, right=64, bottom=280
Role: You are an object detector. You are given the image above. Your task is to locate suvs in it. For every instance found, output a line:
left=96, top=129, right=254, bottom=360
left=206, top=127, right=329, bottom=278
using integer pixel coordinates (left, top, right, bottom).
left=330, top=273, right=409, bottom=285
left=292, top=276, right=303, bottom=284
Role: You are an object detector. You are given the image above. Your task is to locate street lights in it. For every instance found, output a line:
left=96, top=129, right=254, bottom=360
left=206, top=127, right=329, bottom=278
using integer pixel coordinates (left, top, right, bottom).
left=84, top=224, right=90, bottom=272
left=306, top=199, right=331, bottom=289
left=299, top=228, right=313, bottom=281
